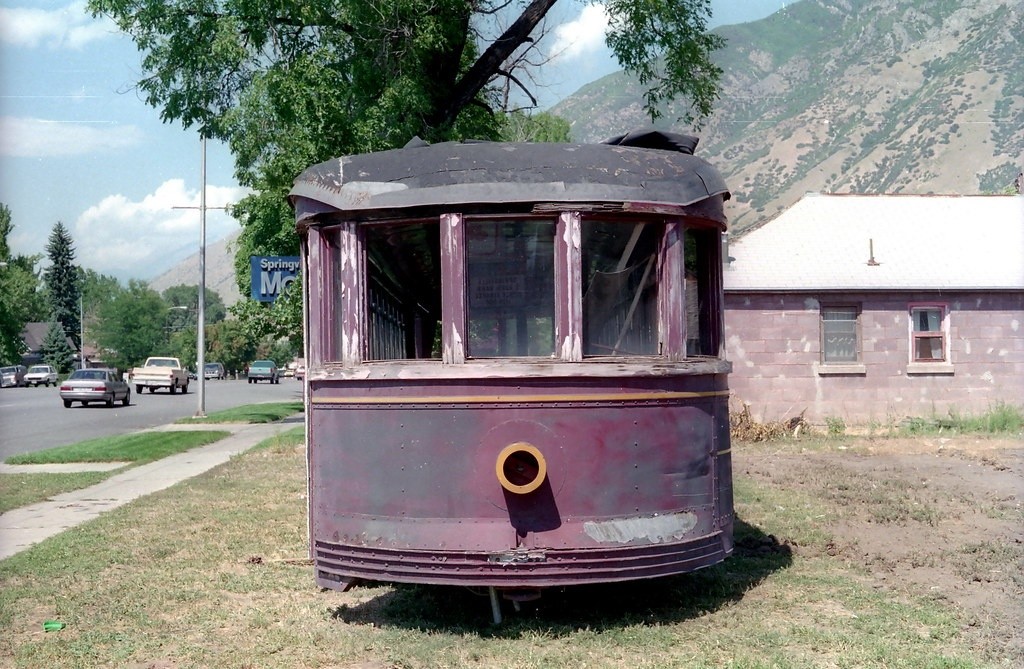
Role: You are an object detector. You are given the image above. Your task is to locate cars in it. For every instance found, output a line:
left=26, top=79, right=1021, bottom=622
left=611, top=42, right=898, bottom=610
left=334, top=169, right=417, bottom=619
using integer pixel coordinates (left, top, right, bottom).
left=295, top=366, right=305, bottom=380
left=188, top=372, right=198, bottom=380
left=60, top=369, right=131, bottom=407
left=278, top=370, right=294, bottom=377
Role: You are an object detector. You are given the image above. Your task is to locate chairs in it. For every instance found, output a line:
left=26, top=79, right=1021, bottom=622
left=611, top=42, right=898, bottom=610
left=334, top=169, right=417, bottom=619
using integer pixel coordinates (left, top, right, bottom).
left=86, top=374, right=93, bottom=378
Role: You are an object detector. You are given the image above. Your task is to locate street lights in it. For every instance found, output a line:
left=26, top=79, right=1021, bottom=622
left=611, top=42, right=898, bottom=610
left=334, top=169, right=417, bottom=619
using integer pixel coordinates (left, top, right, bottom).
left=165, top=306, right=187, bottom=331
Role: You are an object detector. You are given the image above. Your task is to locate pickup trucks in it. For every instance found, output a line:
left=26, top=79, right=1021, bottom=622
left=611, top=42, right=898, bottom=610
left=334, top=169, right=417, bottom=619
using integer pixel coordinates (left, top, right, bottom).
left=247, top=361, right=279, bottom=384
left=132, top=357, right=190, bottom=395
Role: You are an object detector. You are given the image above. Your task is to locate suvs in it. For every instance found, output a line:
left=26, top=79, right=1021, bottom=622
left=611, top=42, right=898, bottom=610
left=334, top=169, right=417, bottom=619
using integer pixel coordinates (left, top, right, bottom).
left=23, top=366, right=58, bottom=387
left=205, top=363, right=225, bottom=380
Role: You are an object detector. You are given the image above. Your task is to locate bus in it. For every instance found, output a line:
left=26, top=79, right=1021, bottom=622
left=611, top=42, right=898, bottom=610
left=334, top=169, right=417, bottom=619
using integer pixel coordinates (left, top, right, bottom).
left=286, top=139, right=733, bottom=603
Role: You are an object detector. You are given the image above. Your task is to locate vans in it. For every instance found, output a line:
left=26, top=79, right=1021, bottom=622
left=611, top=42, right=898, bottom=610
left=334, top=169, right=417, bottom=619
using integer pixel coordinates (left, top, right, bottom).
left=0, top=365, right=29, bottom=387
